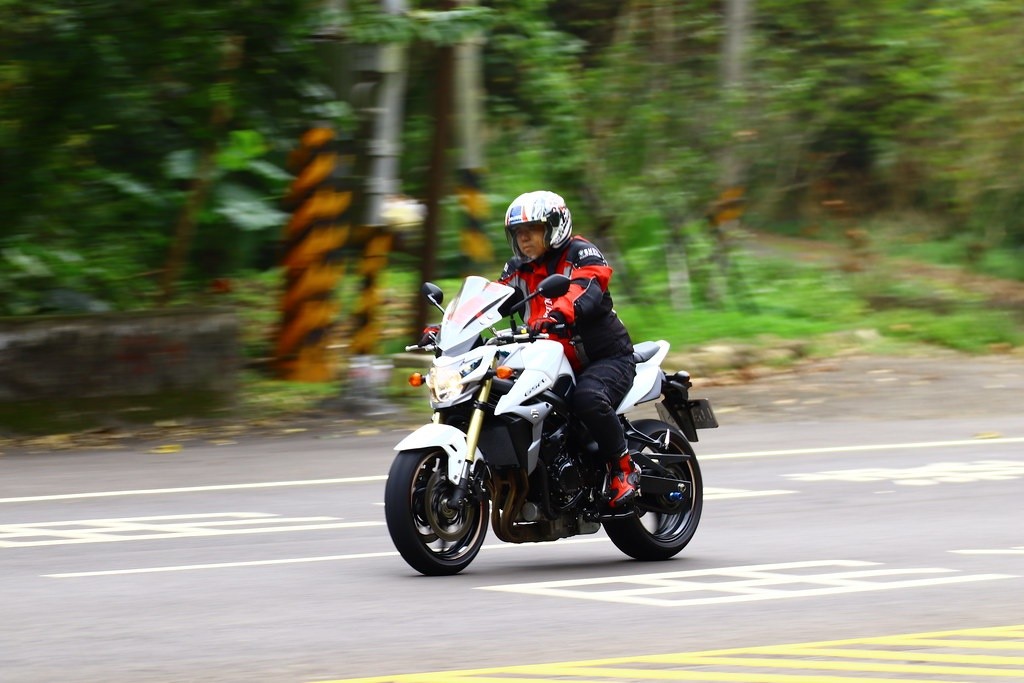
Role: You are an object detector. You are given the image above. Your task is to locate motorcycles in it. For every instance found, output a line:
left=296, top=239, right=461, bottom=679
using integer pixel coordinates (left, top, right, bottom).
left=384, top=273, right=719, bottom=576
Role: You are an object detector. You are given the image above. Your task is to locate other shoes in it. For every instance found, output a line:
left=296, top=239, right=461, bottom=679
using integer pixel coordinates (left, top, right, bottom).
left=607, top=448, right=642, bottom=508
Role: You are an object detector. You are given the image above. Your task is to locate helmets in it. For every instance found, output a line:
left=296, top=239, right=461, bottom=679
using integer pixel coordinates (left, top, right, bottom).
left=505, top=191, right=572, bottom=264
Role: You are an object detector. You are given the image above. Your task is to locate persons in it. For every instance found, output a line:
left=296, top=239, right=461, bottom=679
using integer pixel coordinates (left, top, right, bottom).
left=418, top=190, right=642, bottom=506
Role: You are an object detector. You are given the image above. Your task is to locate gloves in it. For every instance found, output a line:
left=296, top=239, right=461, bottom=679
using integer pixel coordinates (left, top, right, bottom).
left=419, top=324, right=439, bottom=347
left=526, top=318, right=558, bottom=343
